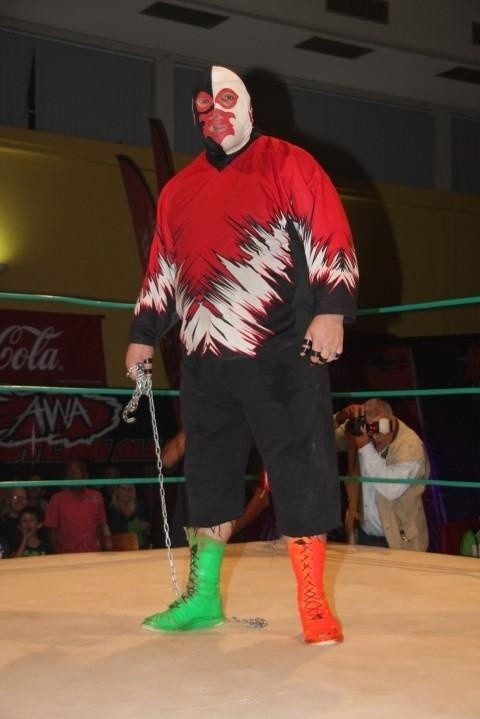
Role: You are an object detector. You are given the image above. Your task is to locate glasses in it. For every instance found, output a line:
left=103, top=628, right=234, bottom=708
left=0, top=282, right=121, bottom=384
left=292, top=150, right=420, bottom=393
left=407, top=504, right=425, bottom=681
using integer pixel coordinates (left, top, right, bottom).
left=10, top=496, right=28, bottom=501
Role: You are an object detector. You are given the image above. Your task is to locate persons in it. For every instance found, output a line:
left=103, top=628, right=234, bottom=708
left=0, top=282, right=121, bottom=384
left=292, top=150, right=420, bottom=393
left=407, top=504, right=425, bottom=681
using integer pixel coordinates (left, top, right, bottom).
left=163, top=426, right=270, bottom=547
left=126, top=64, right=361, bottom=649
left=459, top=505, right=480, bottom=557
left=1, top=454, right=154, bottom=558
left=332, top=399, right=431, bottom=553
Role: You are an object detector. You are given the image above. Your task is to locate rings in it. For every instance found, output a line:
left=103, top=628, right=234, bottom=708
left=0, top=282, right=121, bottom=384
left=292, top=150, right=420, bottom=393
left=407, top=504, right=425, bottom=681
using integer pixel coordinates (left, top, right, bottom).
left=309, top=359, right=318, bottom=366
left=336, top=353, right=342, bottom=358
left=318, top=363, right=324, bottom=368
left=311, top=350, right=321, bottom=357
left=300, top=346, right=309, bottom=354
left=319, top=356, right=327, bottom=362
left=144, top=369, right=153, bottom=374
left=302, top=339, right=313, bottom=346
left=143, top=358, right=152, bottom=363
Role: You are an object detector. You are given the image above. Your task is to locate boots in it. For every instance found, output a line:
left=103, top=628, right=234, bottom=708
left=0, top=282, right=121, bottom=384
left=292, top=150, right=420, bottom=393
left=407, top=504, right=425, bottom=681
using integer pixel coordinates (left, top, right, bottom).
left=139, top=534, right=227, bottom=634
left=286, top=536, right=345, bottom=649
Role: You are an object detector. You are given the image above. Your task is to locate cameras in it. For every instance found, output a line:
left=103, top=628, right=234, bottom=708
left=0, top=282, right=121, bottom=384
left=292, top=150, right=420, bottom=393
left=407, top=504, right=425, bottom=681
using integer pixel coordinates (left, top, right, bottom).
left=346, top=412, right=366, bottom=435
